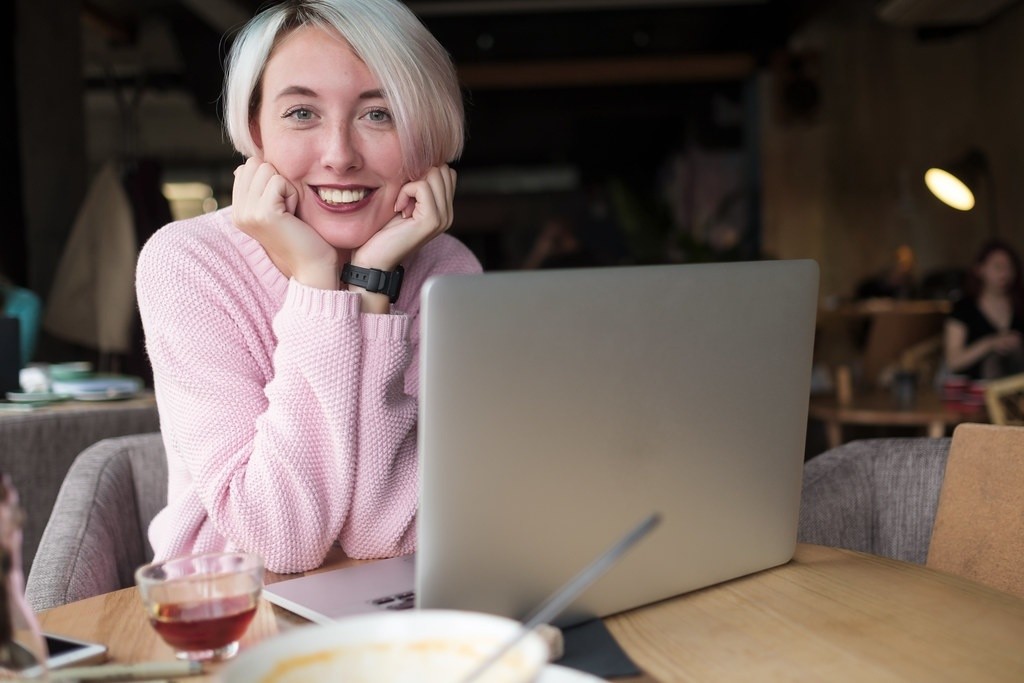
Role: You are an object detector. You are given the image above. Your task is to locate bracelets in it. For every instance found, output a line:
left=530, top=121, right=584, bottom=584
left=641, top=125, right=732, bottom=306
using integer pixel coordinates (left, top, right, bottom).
left=340, top=260, right=404, bottom=304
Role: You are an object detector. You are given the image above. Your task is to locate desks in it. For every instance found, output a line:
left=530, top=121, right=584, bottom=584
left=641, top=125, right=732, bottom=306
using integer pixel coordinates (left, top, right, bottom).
left=0, top=388, right=162, bottom=671
left=33, top=541, right=1024, bottom=683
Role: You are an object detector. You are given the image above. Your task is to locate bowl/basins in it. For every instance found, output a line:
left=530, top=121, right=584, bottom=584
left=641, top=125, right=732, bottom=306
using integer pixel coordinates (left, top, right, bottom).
left=135, top=550, right=262, bottom=663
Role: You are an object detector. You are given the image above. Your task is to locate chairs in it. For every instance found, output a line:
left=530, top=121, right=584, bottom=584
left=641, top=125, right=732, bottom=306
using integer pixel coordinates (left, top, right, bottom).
left=926, top=421, right=1024, bottom=597
left=19, top=429, right=169, bottom=615
left=795, top=436, right=952, bottom=564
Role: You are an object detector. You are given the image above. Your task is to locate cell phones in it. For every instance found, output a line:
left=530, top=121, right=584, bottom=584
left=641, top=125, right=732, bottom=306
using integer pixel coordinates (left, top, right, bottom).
left=15, top=631, right=107, bottom=680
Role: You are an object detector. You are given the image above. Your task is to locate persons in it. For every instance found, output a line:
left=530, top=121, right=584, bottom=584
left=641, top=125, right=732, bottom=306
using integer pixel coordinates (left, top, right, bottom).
left=848, top=243, right=922, bottom=353
left=135, top=1, right=490, bottom=573
left=940, top=240, right=1024, bottom=390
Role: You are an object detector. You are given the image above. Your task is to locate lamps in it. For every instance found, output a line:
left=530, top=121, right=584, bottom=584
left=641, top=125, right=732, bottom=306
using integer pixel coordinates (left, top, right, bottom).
left=922, top=143, right=992, bottom=213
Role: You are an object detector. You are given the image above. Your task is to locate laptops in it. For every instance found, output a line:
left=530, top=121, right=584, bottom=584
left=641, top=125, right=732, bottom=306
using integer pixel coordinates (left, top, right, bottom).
left=261, top=260, right=817, bottom=625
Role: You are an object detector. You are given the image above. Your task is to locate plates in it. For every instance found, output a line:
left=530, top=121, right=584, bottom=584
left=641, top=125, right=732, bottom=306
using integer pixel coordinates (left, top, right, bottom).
left=215, top=607, right=551, bottom=683
left=7, top=391, right=73, bottom=405
left=534, top=664, right=614, bottom=683
left=74, top=386, right=135, bottom=400
